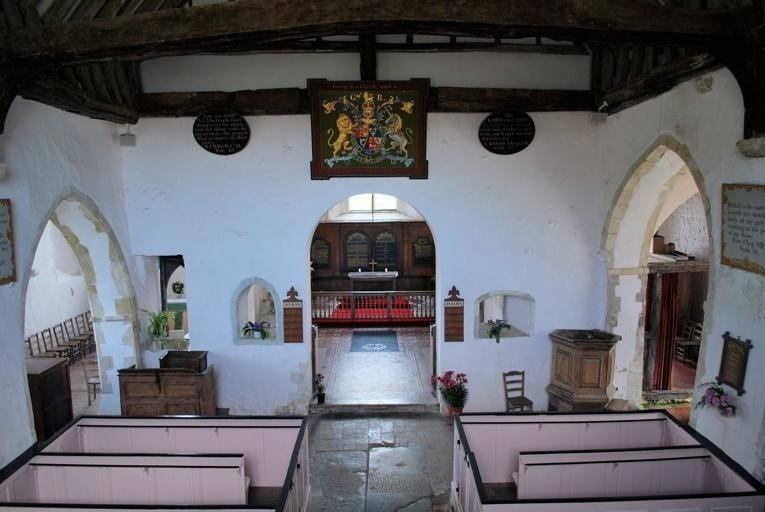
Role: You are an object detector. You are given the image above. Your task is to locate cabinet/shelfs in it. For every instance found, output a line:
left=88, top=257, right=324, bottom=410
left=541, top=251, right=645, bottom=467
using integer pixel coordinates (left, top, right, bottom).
left=24, top=356, right=74, bottom=443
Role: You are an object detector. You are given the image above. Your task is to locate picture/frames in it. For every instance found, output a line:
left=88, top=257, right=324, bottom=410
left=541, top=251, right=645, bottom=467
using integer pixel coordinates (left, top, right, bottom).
left=713, top=329, right=754, bottom=397
left=304, top=76, right=430, bottom=182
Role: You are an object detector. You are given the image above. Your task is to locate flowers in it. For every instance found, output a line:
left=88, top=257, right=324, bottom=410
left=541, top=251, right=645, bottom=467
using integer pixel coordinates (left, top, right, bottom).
left=690, top=379, right=737, bottom=422
left=240, top=320, right=271, bottom=340
left=312, top=372, right=326, bottom=393
left=433, top=369, right=469, bottom=409
left=486, top=317, right=512, bottom=343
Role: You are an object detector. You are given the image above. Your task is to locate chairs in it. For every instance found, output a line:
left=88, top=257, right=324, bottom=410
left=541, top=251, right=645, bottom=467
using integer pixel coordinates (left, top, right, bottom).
left=502, top=369, right=533, bottom=412
left=674, top=317, right=704, bottom=365
left=674, top=318, right=696, bottom=361
left=21, top=309, right=100, bottom=408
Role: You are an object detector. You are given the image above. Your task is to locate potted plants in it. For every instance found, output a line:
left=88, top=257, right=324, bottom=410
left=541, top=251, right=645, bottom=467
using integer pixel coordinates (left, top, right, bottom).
left=141, top=305, right=172, bottom=350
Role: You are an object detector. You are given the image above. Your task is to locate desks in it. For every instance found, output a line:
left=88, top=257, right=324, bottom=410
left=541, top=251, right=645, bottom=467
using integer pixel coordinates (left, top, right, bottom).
left=347, top=270, right=401, bottom=311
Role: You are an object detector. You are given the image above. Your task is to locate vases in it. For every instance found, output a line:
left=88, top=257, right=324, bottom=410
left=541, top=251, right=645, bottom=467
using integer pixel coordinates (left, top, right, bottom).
left=446, top=403, right=464, bottom=426
left=316, top=391, right=326, bottom=404
left=250, top=330, right=262, bottom=338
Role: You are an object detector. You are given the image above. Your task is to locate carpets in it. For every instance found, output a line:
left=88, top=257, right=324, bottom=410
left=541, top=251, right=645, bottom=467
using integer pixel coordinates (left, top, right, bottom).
left=331, top=297, right=414, bottom=318
left=349, top=329, right=400, bottom=353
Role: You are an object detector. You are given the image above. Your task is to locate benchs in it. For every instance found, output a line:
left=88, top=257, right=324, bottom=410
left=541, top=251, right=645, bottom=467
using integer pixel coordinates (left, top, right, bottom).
left=509, top=442, right=712, bottom=501
left=29, top=448, right=251, bottom=508
